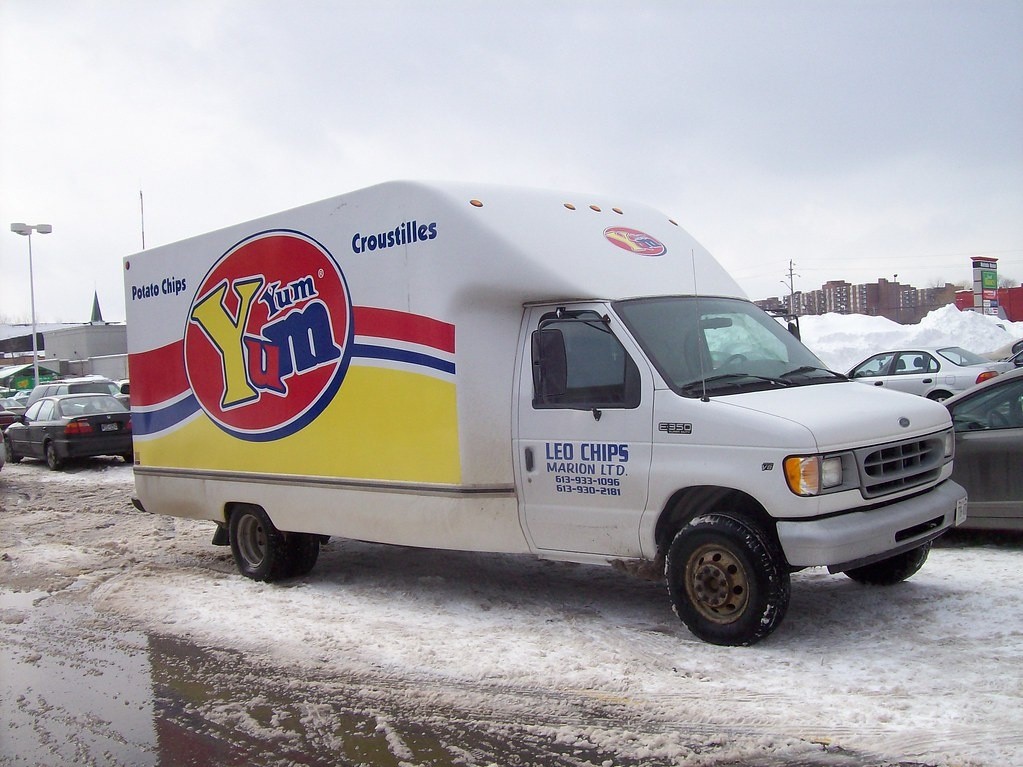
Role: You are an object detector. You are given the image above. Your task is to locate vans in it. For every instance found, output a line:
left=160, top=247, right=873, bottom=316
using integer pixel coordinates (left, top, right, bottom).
left=121, top=177, right=968, bottom=648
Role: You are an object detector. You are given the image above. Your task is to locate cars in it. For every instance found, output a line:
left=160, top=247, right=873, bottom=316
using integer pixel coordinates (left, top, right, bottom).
left=976, top=337, right=1023, bottom=369
left=938, top=366, right=1023, bottom=535
left=837, top=344, right=1019, bottom=402
left=3, top=393, right=133, bottom=472
left=0, top=375, right=130, bottom=472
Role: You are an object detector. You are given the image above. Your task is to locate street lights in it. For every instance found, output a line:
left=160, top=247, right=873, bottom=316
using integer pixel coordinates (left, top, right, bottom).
left=11, top=223, right=53, bottom=387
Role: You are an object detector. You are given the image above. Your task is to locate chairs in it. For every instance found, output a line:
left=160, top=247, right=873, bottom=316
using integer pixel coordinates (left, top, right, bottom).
left=896, top=359, right=906, bottom=370
left=914, top=358, right=923, bottom=368
